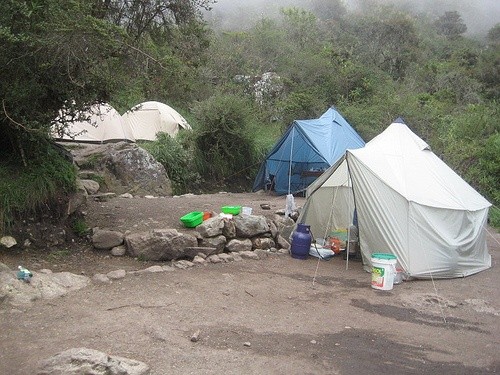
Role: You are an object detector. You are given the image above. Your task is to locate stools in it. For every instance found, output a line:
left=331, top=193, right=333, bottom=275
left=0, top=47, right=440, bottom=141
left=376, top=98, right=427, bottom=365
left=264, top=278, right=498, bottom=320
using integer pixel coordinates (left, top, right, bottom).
left=289, top=182, right=306, bottom=197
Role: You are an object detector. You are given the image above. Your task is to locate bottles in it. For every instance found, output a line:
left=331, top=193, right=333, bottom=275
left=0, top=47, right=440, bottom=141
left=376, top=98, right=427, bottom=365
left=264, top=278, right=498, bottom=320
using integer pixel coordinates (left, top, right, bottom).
left=18, top=264, right=33, bottom=279
left=325, top=236, right=338, bottom=257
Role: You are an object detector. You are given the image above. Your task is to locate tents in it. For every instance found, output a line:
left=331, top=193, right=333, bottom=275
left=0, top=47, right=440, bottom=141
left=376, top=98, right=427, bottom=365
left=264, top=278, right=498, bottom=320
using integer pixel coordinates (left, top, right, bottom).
left=252, top=108, right=366, bottom=199
left=49, top=99, right=134, bottom=142
left=288, top=119, right=492, bottom=278
left=120, top=101, right=193, bottom=142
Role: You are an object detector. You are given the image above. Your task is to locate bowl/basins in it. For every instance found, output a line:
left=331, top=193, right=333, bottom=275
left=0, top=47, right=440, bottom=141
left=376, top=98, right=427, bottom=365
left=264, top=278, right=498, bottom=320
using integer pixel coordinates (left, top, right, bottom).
left=180, top=203, right=241, bottom=228
left=260, top=203, right=270, bottom=209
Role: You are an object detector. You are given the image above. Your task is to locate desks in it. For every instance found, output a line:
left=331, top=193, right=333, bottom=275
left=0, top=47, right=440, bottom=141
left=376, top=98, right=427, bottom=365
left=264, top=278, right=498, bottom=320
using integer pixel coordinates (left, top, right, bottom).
left=299, top=170, right=322, bottom=196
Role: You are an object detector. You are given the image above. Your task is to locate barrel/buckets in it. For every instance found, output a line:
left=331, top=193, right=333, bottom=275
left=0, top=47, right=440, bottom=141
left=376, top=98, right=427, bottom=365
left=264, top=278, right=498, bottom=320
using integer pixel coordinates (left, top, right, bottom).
left=346, top=239, right=358, bottom=256
left=371, top=254, right=397, bottom=290
left=392, top=268, right=402, bottom=285
